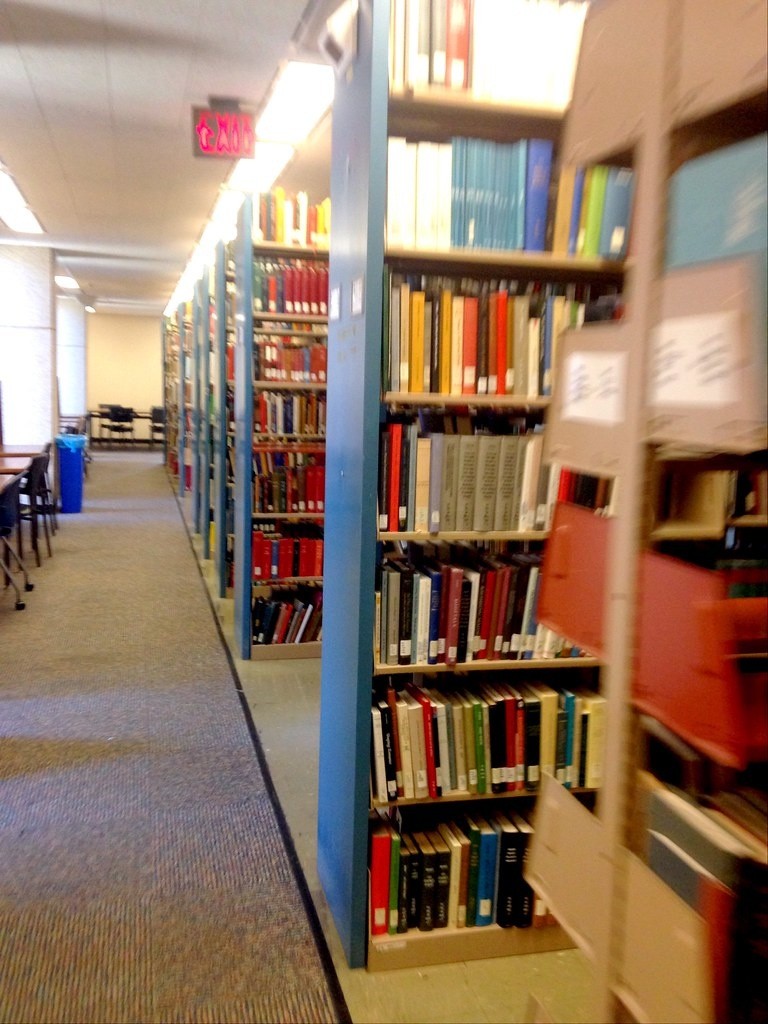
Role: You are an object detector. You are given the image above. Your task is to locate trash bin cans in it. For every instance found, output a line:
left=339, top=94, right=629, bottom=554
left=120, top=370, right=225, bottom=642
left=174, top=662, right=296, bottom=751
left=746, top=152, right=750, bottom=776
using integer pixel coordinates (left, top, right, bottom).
left=55, top=434, right=86, bottom=513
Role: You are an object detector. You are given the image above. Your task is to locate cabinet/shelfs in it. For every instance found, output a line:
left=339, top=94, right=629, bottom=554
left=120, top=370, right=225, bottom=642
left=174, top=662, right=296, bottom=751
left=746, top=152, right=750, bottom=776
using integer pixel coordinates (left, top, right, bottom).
left=159, top=0, right=768, bottom=1024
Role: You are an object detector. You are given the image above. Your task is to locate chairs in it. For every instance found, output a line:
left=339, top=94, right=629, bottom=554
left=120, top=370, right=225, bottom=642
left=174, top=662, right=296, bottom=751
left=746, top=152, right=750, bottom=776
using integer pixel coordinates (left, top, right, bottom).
left=0, top=443, right=58, bottom=608
left=99, top=407, right=136, bottom=451
left=150, top=407, right=166, bottom=449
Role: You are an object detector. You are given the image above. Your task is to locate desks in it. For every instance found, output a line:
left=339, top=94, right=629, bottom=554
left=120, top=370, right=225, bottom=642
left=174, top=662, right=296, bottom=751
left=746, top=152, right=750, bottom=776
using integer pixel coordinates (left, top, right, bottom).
left=0, top=455, right=34, bottom=475
left=0, top=444, right=45, bottom=457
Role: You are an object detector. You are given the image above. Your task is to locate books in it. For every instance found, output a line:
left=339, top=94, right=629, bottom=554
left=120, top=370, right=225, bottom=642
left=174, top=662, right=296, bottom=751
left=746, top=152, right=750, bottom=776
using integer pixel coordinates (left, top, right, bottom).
left=665, top=129, right=768, bottom=435
left=254, top=320, right=330, bottom=382
left=388, top=0, right=478, bottom=91
left=254, top=254, right=332, bottom=314
left=626, top=713, right=768, bottom=1024
left=379, top=412, right=562, bottom=534
left=370, top=678, right=607, bottom=804
left=166, top=241, right=237, bottom=594
left=368, top=813, right=566, bottom=935
left=388, top=135, right=631, bottom=259
left=252, top=190, right=332, bottom=248
left=643, top=458, right=768, bottom=677
left=250, top=390, right=328, bottom=441
left=252, top=451, right=326, bottom=513
left=251, top=520, right=328, bottom=590
left=383, top=263, right=623, bottom=395
left=253, top=593, right=323, bottom=644
left=374, top=539, right=601, bottom=667
